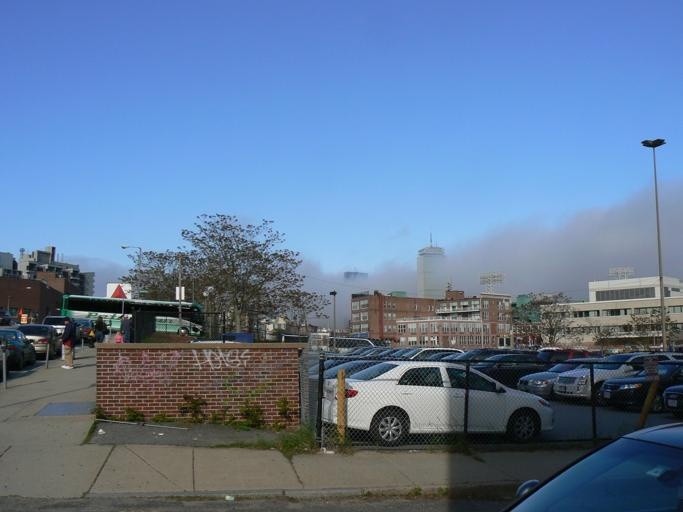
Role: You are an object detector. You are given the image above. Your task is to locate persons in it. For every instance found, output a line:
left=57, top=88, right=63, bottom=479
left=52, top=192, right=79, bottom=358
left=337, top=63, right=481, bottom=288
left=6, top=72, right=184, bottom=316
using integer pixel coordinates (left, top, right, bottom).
left=16, top=311, right=133, bottom=348
left=59, top=316, right=77, bottom=369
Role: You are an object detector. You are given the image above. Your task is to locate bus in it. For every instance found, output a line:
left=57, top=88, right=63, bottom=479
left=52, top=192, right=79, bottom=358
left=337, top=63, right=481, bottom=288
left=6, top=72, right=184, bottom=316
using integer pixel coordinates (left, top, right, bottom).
left=60, top=295, right=206, bottom=338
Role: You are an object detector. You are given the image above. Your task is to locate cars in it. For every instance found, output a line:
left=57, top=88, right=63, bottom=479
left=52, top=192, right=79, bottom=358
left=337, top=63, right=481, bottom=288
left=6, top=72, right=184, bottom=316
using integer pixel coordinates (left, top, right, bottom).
left=501, top=416, right=683, bottom=512
left=0, top=315, right=96, bottom=373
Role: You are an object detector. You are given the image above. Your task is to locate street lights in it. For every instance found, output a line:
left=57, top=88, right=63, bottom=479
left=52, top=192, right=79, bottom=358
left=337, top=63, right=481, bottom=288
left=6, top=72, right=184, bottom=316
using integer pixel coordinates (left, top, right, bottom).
left=328, top=290, right=338, bottom=353
left=639, top=137, right=669, bottom=352
left=121, top=245, right=141, bottom=299
left=202, top=286, right=216, bottom=338
